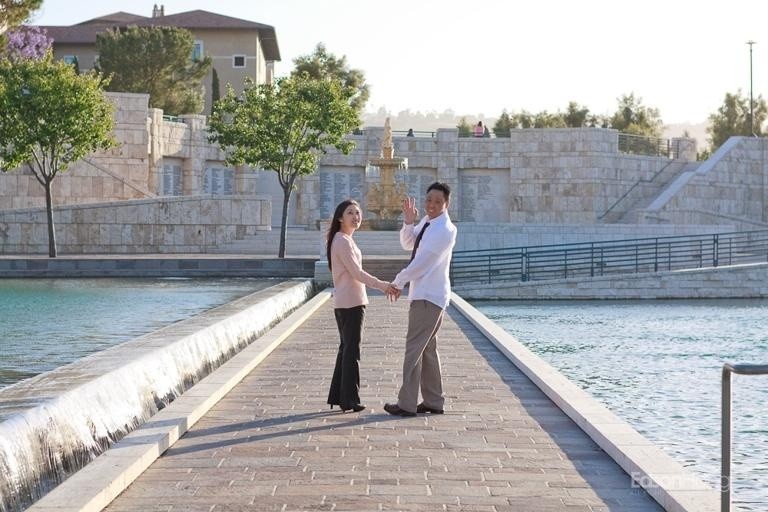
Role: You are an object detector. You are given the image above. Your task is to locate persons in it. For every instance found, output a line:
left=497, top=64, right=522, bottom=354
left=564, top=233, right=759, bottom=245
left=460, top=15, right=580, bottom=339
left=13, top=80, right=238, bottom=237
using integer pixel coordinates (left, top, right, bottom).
left=407, top=128, right=414, bottom=136
left=327, top=199, right=396, bottom=413
left=383, top=181, right=459, bottom=416
left=474, top=120, right=485, bottom=137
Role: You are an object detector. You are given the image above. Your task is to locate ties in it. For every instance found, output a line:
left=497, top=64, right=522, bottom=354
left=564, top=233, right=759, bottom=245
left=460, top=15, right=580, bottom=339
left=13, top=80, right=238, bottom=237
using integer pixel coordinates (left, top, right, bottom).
left=407, top=222, right=430, bottom=263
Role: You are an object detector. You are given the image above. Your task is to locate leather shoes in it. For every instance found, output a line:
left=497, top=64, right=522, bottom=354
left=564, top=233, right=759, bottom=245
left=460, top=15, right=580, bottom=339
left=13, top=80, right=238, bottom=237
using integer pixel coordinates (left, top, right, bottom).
left=417, top=403, right=444, bottom=414
left=384, top=403, right=417, bottom=417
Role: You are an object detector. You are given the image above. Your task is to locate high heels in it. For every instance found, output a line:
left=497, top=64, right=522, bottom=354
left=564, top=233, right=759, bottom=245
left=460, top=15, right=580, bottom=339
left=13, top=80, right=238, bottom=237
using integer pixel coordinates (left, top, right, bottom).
left=330, top=402, right=365, bottom=413
